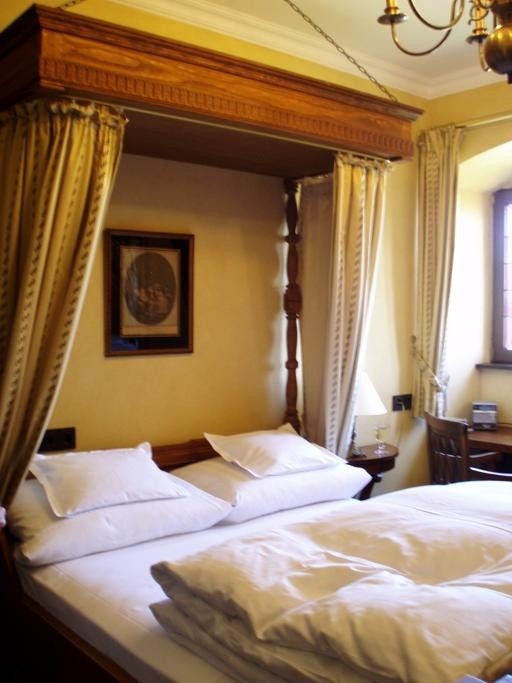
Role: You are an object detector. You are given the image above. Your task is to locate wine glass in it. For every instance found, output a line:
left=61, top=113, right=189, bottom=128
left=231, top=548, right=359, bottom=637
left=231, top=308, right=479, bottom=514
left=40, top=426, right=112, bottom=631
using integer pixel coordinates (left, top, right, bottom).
left=372, top=423, right=391, bottom=456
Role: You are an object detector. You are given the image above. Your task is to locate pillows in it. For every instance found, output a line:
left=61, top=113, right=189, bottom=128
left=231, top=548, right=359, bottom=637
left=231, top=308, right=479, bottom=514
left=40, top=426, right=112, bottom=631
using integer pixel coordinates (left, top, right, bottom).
left=168, top=426, right=370, bottom=526
left=9, top=442, right=233, bottom=566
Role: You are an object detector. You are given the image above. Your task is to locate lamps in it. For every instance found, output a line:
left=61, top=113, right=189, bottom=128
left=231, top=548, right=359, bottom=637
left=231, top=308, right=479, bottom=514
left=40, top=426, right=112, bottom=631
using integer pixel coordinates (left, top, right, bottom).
left=345, top=366, right=389, bottom=458
left=376, top=0, right=512, bottom=85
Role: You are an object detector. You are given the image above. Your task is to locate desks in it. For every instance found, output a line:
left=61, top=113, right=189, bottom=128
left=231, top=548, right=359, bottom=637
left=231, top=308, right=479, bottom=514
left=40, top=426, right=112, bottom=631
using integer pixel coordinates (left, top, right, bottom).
left=344, top=443, right=398, bottom=499
left=462, top=423, right=512, bottom=471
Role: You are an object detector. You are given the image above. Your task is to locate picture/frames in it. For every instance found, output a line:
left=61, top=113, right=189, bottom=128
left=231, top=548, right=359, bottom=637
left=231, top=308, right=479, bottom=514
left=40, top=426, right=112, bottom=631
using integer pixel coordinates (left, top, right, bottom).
left=99, top=225, right=195, bottom=356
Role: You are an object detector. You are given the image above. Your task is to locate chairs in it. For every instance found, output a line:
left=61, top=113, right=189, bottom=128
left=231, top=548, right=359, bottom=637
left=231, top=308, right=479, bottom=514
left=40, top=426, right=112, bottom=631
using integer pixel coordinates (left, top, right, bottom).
left=422, top=410, right=511, bottom=482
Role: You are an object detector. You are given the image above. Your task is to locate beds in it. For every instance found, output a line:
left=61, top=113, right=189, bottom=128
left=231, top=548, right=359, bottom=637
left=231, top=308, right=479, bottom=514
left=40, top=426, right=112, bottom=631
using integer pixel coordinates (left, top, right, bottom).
left=0, top=423, right=510, bottom=682
